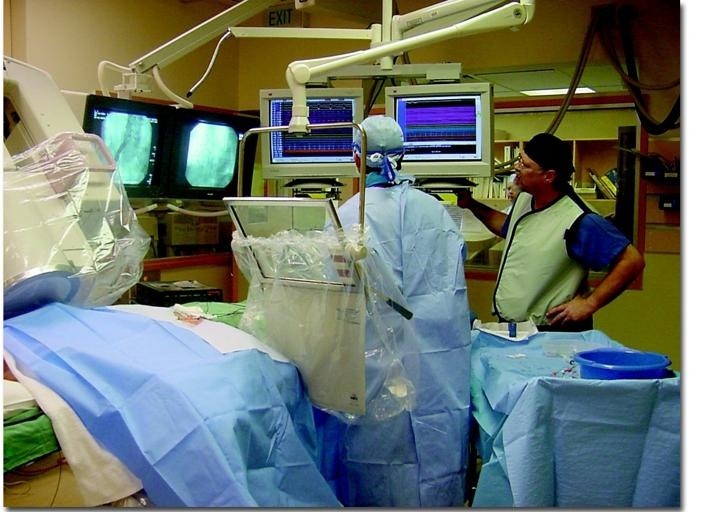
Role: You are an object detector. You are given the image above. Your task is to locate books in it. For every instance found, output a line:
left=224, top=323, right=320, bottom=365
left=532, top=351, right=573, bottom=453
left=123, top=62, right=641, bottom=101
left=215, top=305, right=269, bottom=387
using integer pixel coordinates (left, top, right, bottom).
left=468, top=144, right=521, bottom=198
left=574, top=168, right=619, bottom=200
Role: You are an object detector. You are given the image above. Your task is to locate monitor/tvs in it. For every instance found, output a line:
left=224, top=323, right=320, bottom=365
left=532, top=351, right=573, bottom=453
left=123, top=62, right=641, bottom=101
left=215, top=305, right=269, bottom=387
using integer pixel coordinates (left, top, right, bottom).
left=385, top=82, right=494, bottom=189
left=166, top=108, right=260, bottom=200
left=260, top=87, right=364, bottom=188
left=83, top=94, right=176, bottom=198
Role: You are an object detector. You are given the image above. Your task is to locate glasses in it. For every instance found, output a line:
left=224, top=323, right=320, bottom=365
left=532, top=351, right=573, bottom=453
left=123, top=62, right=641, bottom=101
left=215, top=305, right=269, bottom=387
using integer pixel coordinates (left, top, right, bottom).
left=517, top=154, right=544, bottom=173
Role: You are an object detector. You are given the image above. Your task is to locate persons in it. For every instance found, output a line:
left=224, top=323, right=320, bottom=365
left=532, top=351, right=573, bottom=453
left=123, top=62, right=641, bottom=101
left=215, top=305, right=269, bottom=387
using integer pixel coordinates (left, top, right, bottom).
left=499, top=173, right=521, bottom=215
left=450, top=131, right=647, bottom=331
left=316, top=113, right=475, bottom=507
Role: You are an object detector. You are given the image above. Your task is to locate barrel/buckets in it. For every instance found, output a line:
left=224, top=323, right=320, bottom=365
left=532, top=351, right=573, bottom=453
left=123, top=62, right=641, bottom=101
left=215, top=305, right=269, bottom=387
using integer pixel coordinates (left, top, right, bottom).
left=576, top=349, right=670, bottom=380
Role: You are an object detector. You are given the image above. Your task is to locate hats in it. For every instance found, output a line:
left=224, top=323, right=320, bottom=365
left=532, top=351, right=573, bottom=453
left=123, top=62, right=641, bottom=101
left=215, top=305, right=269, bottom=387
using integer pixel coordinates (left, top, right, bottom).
left=524, top=133, right=575, bottom=182
left=353, top=114, right=405, bottom=169
